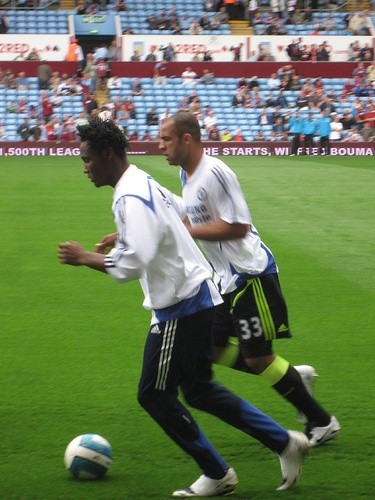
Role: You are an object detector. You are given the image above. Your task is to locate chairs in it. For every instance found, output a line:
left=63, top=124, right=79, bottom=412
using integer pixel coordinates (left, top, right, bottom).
left=0, top=77, right=375, bottom=143
left=0, top=0, right=375, bottom=36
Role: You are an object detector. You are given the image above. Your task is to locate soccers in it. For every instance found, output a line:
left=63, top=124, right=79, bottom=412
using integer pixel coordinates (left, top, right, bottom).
left=64, top=434, right=114, bottom=480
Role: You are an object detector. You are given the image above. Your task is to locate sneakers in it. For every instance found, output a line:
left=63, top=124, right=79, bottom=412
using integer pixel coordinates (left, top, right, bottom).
left=172, top=466, right=239, bottom=498
left=308, top=415, right=341, bottom=449
left=276, top=430, right=309, bottom=493
left=292, top=365, right=319, bottom=424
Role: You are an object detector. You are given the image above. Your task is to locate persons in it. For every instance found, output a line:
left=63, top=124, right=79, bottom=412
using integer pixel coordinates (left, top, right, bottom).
left=159, top=110, right=341, bottom=450
left=58, top=120, right=311, bottom=498
left=0, top=1, right=375, bottom=156
